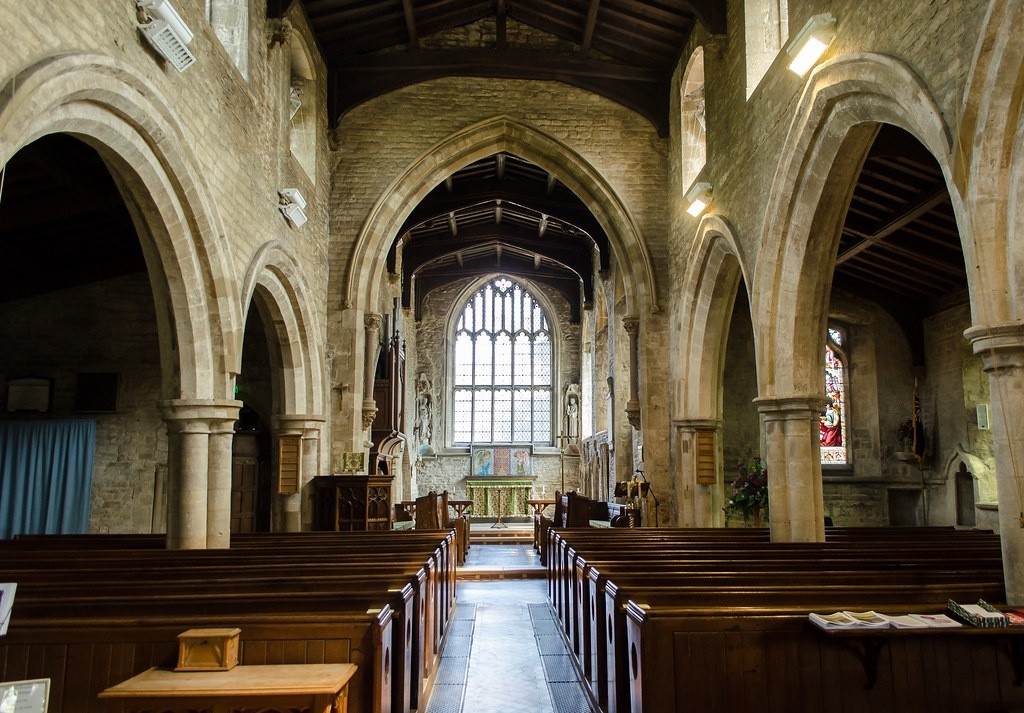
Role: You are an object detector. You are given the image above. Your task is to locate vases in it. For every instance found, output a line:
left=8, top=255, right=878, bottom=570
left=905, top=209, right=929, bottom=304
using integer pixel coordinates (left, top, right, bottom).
left=753, top=502, right=762, bottom=528
left=903, top=437, right=911, bottom=453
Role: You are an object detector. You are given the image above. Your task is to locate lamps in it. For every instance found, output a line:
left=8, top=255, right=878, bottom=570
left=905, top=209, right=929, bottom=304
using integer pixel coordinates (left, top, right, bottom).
left=786, top=11, right=837, bottom=78
left=686, top=182, right=715, bottom=218
left=277, top=185, right=308, bottom=229
left=135, top=0, right=197, bottom=73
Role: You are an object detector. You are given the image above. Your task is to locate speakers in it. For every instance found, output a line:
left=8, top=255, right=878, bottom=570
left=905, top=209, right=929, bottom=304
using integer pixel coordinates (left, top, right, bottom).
left=976, top=404, right=990, bottom=429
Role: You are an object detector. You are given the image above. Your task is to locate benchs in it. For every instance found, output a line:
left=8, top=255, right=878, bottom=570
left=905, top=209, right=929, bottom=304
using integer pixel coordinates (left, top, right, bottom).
left=546, top=527, right=1024, bottom=713
left=0, top=529, right=459, bottom=713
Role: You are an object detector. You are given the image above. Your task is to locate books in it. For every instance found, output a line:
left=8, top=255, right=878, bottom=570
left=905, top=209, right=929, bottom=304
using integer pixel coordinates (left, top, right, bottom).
left=809, top=611, right=962, bottom=629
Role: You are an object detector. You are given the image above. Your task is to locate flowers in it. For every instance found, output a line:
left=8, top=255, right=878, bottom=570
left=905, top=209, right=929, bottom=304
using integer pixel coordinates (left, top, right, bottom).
left=720, top=457, right=770, bottom=522
left=896, top=419, right=915, bottom=439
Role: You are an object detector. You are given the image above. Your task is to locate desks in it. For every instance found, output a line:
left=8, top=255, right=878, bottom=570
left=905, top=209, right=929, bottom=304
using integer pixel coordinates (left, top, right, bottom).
left=97, top=664, right=358, bottom=713
left=313, top=475, right=396, bottom=531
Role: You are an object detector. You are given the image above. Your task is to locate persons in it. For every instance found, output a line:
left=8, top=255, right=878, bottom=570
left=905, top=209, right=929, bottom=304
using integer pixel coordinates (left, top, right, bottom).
left=420, top=408, right=430, bottom=445
left=567, top=398, right=577, bottom=436
